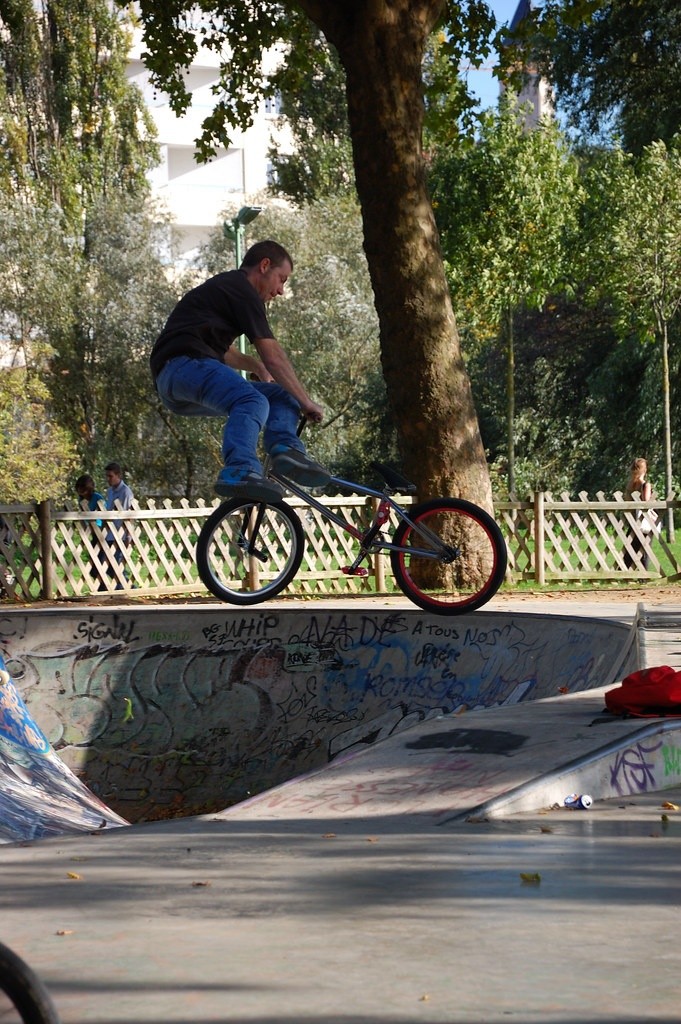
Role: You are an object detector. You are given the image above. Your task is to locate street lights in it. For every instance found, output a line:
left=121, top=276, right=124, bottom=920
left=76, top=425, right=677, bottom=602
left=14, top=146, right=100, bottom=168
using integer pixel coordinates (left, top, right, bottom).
left=223, top=204, right=265, bottom=378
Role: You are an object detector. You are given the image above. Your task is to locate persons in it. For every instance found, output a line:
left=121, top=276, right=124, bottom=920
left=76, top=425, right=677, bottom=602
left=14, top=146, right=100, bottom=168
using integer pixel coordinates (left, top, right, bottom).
left=617, top=458, right=659, bottom=577
left=73, top=463, right=134, bottom=589
left=150, top=240, right=332, bottom=503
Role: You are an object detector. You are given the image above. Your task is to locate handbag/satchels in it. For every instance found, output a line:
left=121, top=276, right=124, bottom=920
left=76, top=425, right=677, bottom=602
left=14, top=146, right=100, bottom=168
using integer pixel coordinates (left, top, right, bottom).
left=604, top=664, right=681, bottom=717
left=635, top=481, right=661, bottom=535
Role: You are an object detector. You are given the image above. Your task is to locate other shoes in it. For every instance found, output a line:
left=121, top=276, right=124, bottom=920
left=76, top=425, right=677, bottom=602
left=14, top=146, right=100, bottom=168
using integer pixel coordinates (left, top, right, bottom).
left=214, top=469, right=284, bottom=503
left=114, top=577, right=133, bottom=590
left=269, top=443, right=332, bottom=487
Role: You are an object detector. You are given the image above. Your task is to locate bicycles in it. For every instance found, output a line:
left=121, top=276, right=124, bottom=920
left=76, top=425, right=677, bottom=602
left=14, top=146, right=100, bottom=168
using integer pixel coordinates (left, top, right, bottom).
left=194, top=373, right=509, bottom=616
